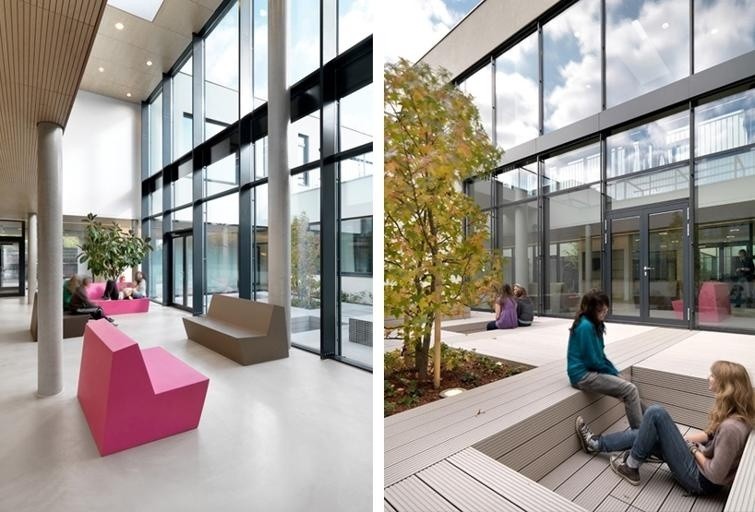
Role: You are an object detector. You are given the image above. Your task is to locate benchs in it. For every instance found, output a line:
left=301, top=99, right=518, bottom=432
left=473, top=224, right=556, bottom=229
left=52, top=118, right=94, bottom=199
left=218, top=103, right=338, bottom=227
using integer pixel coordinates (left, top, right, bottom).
left=527, top=281, right=583, bottom=315
left=32, top=279, right=290, bottom=456
left=672, top=281, right=729, bottom=323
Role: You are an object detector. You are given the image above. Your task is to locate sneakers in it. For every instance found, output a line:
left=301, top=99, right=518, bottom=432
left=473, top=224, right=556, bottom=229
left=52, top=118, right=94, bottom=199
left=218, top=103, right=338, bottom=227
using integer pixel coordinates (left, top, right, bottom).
left=576, top=417, right=597, bottom=454
left=611, top=454, right=639, bottom=484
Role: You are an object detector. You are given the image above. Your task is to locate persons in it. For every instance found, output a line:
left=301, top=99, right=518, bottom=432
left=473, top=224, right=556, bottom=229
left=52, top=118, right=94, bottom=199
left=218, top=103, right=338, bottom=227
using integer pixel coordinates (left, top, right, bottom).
left=734, top=249, right=753, bottom=308
left=575, top=360, right=755, bottom=497
left=513, top=285, right=533, bottom=327
left=132, top=272, right=147, bottom=299
left=63, top=276, right=79, bottom=310
left=102, top=280, right=138, bottom=301
left=69, top=277, right=114, bottom=322
left=567, top=288, right=667, bottom=462
left=487, top=284, right=518, bottom=330
